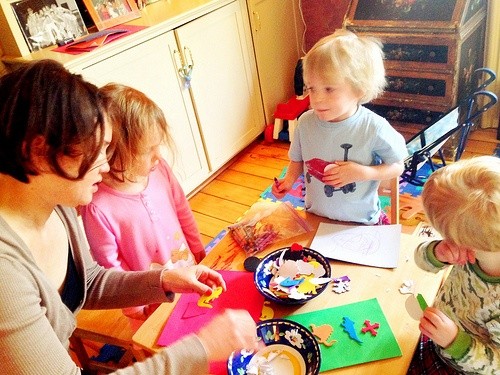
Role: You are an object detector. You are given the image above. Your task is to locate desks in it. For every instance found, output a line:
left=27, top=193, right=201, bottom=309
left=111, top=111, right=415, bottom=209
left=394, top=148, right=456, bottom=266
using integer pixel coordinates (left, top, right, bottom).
left=131, top=197, right=448, bottom=375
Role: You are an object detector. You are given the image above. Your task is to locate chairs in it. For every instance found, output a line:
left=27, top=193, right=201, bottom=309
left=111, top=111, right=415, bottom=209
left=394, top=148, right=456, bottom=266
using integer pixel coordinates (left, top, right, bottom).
left=376, top=175, right=401, bottom=225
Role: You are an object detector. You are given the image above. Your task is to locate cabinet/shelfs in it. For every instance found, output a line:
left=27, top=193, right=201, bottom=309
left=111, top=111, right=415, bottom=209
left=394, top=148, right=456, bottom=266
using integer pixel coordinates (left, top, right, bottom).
left=0, top=0, right=488, bottom=201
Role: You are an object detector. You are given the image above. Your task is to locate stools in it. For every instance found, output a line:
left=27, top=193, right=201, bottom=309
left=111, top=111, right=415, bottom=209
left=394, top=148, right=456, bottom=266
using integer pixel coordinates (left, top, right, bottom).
left=68, top=306, right=151, bottom=374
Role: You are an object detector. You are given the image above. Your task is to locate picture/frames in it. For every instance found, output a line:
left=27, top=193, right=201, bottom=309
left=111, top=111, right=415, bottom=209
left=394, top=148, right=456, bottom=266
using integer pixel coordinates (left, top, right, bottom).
left=82, top=0, right=143, bottom=31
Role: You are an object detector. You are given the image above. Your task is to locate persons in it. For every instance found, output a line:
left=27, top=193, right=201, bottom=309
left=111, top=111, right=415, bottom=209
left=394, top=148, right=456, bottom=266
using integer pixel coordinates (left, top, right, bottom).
left=413, top=154, right=500, bottom=375
left=271, top=28, right=409, bottom=223
left=0, top=59, right=260, bottom=375
left=79, top=82, right=206, bottom=333
left=23, top=3, right=81, bottom=48
left=94, top=0, right=131, bottom=24
